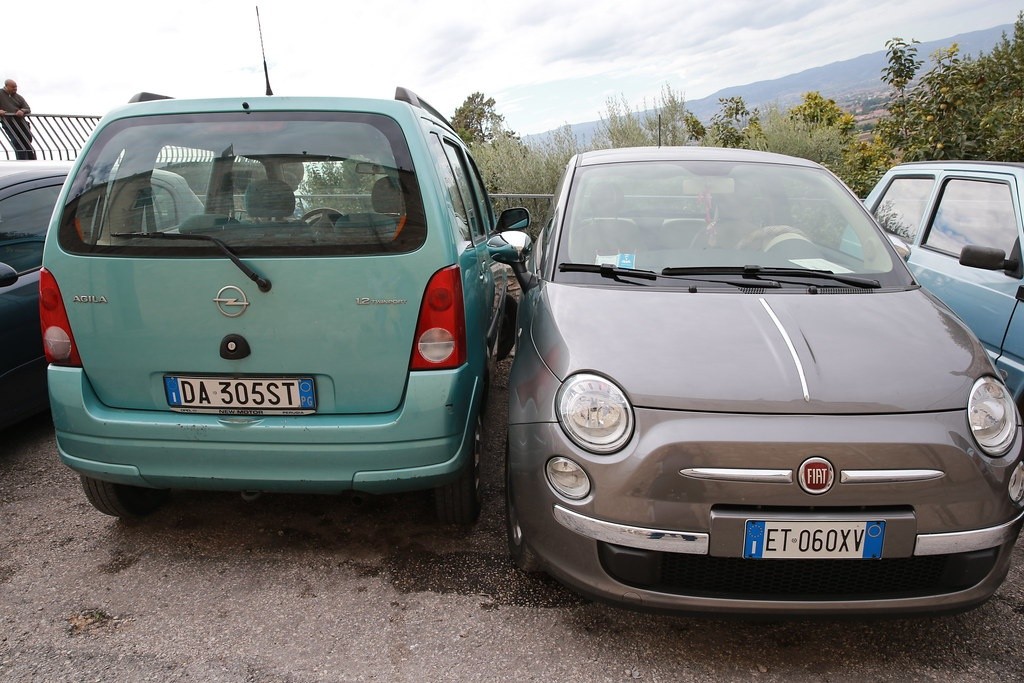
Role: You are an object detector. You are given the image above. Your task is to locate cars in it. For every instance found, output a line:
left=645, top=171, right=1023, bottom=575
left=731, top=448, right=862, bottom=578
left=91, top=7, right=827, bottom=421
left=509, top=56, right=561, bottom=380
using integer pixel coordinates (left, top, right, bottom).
left=486, top=145, right=1024, bottom=621
left=0, top=159, right=204, bottom=433
left=838, top=158, right=1024, bottom=404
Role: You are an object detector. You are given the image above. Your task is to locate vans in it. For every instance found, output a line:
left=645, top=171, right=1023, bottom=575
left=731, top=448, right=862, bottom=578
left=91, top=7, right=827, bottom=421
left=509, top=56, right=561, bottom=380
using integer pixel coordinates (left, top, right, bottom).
left=39, top=85, right=531, bottom=536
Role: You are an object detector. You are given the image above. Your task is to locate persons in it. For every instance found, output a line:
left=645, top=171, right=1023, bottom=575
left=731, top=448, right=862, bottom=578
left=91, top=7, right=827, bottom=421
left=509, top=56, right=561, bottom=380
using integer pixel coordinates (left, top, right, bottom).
left=0, top=79, right=36, bottom=160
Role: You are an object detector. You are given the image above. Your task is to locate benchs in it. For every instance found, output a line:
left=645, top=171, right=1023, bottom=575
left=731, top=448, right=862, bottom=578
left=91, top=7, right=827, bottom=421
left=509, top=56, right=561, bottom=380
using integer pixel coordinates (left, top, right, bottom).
left=183, top=213, right=395, bottom=248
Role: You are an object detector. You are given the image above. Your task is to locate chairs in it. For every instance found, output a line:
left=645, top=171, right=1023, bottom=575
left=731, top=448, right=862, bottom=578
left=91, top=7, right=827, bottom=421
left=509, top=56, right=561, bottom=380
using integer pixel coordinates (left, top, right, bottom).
left=710, top=166, right=786, bottom=252
left=370, top=175, right=407, bottom=232
left=245, top=178, right=300, bottom=225
left=570, top=183, right=641, bottom=261
